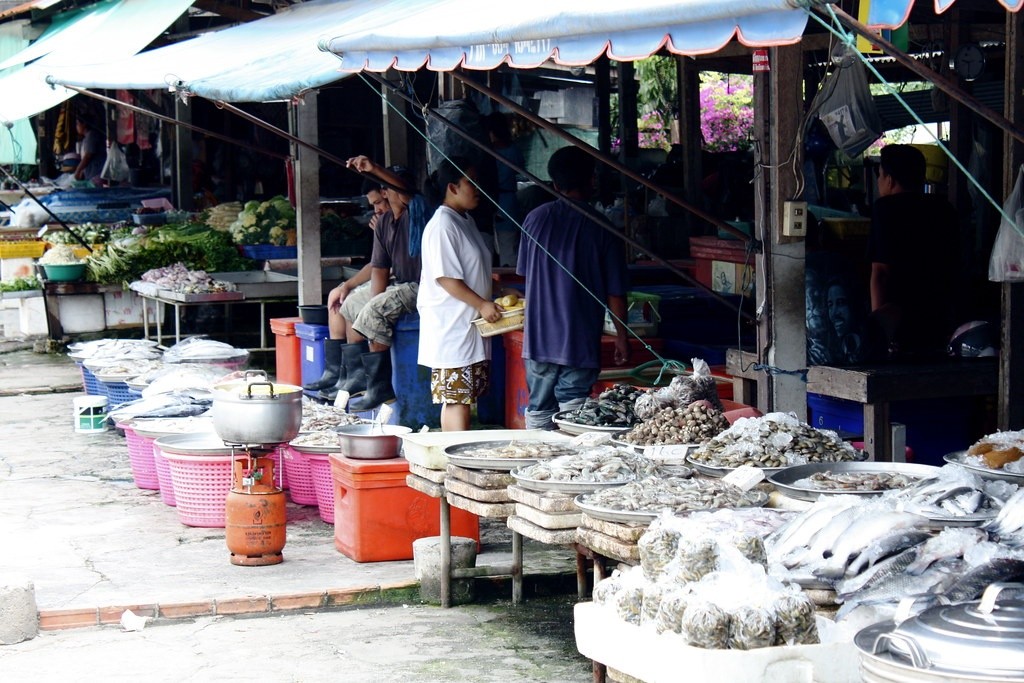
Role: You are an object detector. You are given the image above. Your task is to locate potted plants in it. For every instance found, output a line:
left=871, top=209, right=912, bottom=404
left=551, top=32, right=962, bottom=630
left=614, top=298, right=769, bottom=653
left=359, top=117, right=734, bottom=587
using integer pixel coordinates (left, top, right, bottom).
left=0, top=275, right=43, bottom=299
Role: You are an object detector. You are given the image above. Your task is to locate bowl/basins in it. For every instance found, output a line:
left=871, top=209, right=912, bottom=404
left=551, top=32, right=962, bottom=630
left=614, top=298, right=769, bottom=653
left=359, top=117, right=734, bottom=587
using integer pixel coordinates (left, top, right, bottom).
left=33, top=261, right=87, bottom=279
left=333, top=423, right=412, bottom=460
left=296, top=304, right=329, bottom=324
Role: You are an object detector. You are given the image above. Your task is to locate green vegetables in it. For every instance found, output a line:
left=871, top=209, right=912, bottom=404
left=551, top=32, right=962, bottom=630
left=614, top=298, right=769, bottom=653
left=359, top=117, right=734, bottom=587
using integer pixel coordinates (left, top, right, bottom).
left=82, top=223, right=264, bottom=284
left=0, top=275, right=42, bottom=292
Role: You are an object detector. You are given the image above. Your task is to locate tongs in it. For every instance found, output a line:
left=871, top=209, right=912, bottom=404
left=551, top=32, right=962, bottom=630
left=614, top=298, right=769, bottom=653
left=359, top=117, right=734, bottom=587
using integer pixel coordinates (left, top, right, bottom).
left=368, top=409, right=384, bottom=435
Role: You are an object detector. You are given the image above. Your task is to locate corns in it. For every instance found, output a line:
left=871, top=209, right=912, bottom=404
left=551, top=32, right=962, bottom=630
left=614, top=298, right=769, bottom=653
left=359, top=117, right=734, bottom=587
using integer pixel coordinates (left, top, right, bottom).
left=486, top=294, right=527, bottom=331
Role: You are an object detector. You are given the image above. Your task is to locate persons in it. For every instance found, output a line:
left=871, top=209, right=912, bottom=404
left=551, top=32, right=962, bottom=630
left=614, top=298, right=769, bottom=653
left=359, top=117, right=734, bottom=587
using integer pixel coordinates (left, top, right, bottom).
left=487, top=112, right=521, bottom=193
left=515, top=146, right=631, bottom=432
left=74, top=112, right=107, bottom=181
left=416, top=155, right=525, bottom=432
left=872, top=145, right=960, bottom=467
left=808, top=262, right=871, bottom=368
left=302, top=156, right=423, bottom=413
left=0, top=163, right=37, bottom=184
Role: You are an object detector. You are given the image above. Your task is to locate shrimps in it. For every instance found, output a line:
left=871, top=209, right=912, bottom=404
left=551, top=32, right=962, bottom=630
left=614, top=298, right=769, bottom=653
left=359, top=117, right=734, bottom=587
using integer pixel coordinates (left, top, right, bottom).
left=581, top=475, right=765, bottom=515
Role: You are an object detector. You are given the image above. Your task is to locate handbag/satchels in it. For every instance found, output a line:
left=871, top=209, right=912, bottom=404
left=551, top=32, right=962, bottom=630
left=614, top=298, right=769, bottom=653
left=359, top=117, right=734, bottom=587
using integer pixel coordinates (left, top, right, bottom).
left=809, top=56, right=884, bottom=159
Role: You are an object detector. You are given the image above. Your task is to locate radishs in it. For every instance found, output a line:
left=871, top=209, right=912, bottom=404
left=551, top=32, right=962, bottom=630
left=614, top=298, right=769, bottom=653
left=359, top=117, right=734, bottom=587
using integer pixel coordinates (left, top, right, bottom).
left=207, top=202, right=243, bottom=231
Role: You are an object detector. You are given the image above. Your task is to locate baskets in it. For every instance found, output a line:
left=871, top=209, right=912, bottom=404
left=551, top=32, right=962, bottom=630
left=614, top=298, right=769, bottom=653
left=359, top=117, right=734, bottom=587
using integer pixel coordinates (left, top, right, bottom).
left=474, top=306, right=525, bottom=336
left=78, top=337, right=335, bottom=528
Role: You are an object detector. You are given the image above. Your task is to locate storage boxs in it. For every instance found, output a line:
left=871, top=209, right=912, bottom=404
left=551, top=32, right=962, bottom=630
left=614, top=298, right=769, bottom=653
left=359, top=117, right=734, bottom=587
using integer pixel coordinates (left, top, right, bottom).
left=807, top=393, right=864, bottom=440
left=104, top=291, right=165, bottom=330
left=328, top=452, right=480, bottom=563
left=46, top=294, right=105, bottom=333
left=689, top=236, right=754, bottom=290
left=270, top=317, right=330, bottom=398
left=600, top=285, right=757, bottom=401
left=573, top=600, right=860, bottom=683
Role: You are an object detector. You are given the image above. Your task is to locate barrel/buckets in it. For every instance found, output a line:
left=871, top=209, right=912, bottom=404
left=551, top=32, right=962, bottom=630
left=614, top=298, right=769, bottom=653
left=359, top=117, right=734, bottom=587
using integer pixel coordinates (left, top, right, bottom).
left=73, top=394, right=109, bottom=434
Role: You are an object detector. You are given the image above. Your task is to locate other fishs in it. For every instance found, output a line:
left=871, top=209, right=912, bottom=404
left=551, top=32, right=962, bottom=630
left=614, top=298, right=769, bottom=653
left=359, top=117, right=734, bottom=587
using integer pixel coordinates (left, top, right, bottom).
left=773, top=476, right=1024, bottom=624
left=476, top=440, right=578, bottom=458
left=99, top=393, right=213, bottom=425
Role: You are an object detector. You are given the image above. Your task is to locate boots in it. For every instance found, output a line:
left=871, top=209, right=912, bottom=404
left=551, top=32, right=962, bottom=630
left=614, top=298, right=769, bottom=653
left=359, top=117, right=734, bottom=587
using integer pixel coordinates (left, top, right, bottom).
left=303, top=339, right=347, bottom=391
left=317, top=342, right=350, bottom=399
left=349, top=348, right=396, bottom=411
left=328, top=342, right=368, bottom=399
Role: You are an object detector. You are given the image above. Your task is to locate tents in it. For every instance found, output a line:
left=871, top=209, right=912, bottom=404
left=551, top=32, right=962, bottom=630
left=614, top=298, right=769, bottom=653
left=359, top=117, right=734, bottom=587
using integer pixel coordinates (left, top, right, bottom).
left=0, top=0, right=1024, bottom=121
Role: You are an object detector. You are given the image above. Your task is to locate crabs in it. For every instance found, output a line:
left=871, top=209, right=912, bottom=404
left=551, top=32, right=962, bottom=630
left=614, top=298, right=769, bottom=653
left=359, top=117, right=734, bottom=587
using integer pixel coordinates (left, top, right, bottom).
left=516, top=450, right=694, bottom=483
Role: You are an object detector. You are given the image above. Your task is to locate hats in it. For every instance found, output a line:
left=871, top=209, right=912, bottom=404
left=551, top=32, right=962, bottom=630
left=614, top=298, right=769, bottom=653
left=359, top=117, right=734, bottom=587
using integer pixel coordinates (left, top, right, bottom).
left=382, top=164, right=408, bottom=188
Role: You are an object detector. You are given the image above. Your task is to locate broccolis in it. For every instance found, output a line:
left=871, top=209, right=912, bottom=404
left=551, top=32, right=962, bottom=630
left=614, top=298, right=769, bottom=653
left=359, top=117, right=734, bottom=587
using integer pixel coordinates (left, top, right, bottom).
left=229, top=201, right=290, bottom=246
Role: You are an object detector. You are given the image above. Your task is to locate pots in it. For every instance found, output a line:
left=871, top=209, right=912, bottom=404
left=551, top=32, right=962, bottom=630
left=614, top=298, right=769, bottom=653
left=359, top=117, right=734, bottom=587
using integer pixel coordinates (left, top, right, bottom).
left=212, top=370, right=304, bottom=442
